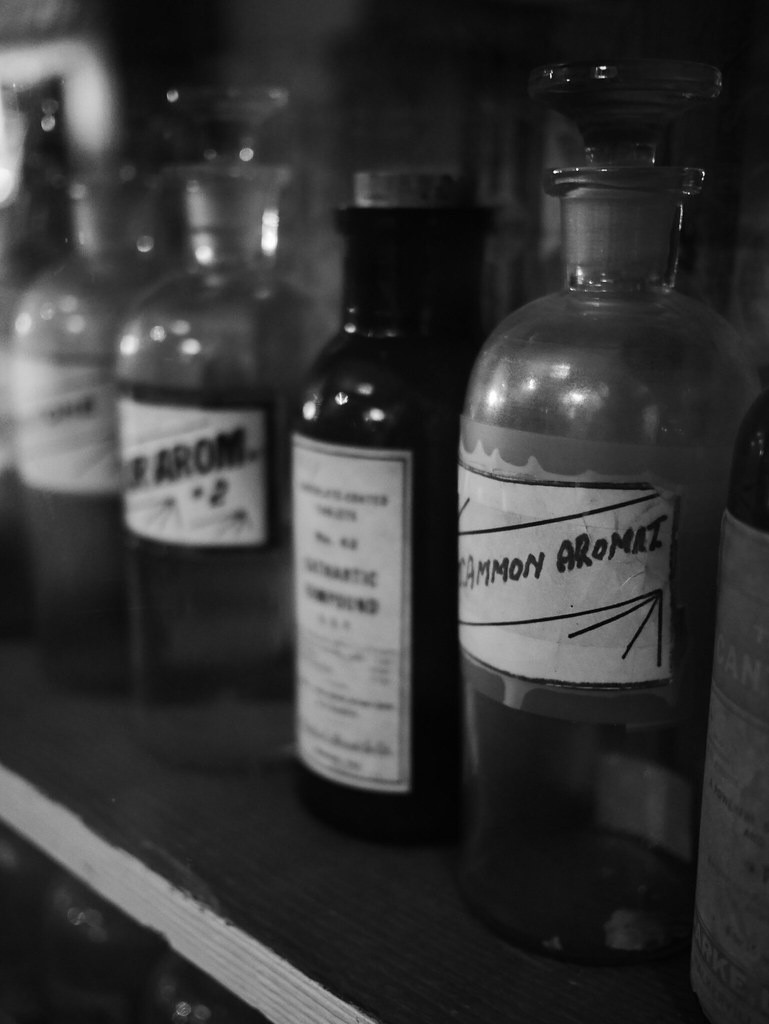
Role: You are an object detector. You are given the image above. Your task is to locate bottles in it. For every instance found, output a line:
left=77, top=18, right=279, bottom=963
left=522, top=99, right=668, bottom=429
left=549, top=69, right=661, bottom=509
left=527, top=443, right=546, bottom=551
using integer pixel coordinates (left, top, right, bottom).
left=0, top=50, right=769, bottom=1024
